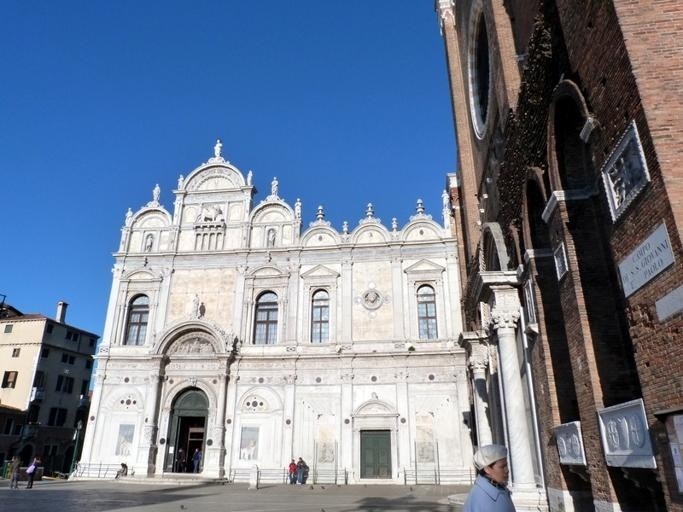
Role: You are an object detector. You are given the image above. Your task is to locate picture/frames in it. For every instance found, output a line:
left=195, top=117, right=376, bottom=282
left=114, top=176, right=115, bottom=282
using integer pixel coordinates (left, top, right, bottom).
left=553, top=241, right=570, bottom=282
left=599, top=118, right=651, bottom=225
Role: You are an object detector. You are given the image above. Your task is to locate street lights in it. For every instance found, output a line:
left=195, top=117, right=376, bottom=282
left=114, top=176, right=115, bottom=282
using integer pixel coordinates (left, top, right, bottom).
left=69, top=421, right=83, bottom=477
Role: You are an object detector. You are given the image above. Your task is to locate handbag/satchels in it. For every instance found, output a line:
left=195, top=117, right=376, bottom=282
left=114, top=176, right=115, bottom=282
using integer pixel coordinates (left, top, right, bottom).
left=25, top=458, right=36, bottom=474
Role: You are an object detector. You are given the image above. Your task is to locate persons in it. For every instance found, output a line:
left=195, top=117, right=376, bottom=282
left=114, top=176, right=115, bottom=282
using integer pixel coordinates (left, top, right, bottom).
left=288, top=458, right=297, bottom=484
left=213, top=138, right=222, bottom=157
left=176, top=173, right=184, bottom=191
left=7, top=454, right=22, bottom=489
left=296, top=456, right=305, bottom=485
left=192, top=292, right=199, bottom=316
left=114, top=462, right=127, bottom=478
left=246, top=170, right=252, bottom=186
left=175, top=447, right=186, bottom=473
left=144, top=235, right=152, bottom=253
left=294, top=197, right=301, bottom=218
left=191, top=448, right=202, bottom=473
left=268, top=229, right=275, bottom=247
left=270, top=176, right=279, bottom=196
left=124, top=207, right=133, bottom=227
left=151, top=183, right=160, bottom=202
left=24, top=456, right=37, bottom=488
left=461, top=444, right=515, bottom=512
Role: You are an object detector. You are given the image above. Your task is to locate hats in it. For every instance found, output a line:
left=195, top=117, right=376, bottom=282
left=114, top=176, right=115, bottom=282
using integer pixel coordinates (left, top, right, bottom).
left=472, top=445, right=507, bottom=471
